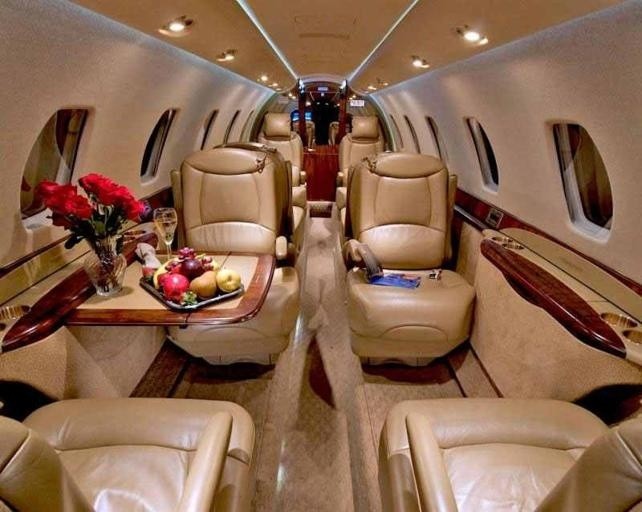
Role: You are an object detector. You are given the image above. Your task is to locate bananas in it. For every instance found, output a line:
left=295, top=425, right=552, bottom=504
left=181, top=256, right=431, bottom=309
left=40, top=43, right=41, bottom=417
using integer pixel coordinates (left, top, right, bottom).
left=153, top=257, right=181, bottom=290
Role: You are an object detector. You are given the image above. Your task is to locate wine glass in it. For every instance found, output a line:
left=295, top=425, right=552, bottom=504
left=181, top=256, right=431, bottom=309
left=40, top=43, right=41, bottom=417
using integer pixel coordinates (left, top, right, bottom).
left=153, top=206, right=178, bottom=261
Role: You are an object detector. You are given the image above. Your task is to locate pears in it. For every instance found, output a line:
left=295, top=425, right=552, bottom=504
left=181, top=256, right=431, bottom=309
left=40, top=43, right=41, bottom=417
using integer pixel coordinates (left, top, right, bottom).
left=190, top=270, right=217, bottom=299
left=216, top=270, right=241, bottom=293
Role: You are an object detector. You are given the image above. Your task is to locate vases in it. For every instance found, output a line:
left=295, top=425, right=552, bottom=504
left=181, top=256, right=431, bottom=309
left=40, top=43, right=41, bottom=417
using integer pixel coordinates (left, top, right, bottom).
left=83, top=246, right=127, bottom=297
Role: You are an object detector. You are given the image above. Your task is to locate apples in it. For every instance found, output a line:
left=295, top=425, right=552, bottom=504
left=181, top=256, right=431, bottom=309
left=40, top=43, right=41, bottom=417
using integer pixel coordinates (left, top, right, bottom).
left=163, top=273, right=189, bottom=300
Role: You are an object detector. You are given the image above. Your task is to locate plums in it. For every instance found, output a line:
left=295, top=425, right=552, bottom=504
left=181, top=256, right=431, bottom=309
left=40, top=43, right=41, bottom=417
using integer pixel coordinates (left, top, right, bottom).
left=180, top=258, right=202, bottom=278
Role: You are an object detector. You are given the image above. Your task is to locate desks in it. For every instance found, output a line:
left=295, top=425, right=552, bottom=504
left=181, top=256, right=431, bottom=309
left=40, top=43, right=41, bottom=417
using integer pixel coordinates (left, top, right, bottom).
left=71, top=250, right=275, bottom=328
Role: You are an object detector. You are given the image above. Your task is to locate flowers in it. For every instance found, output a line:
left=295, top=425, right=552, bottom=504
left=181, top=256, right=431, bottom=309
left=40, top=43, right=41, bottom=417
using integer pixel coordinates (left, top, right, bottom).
left=38, top=173, right=153, bottom=250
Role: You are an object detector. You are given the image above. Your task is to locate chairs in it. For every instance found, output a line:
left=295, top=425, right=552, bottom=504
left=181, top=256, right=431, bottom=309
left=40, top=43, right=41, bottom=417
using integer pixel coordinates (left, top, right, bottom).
left=342, top=152, right=477, bottom=371
left=164, top=148, right=300, bottom=367
left=1, top=395, right=257, bottom=509
left=378, top=397, right=641, bottom=512
left=329, top=112, right=399, bottom=246
left=213, top=110, right=315, bottom=269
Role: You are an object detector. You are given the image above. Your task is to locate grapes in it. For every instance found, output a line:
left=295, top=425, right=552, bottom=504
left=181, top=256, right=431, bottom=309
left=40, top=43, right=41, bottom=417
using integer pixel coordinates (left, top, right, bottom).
left=180, top=247, right=197, bottom=258
left=201, top=256, right=213, bottom=272
left=165, top=261, right=179, bottom=271
